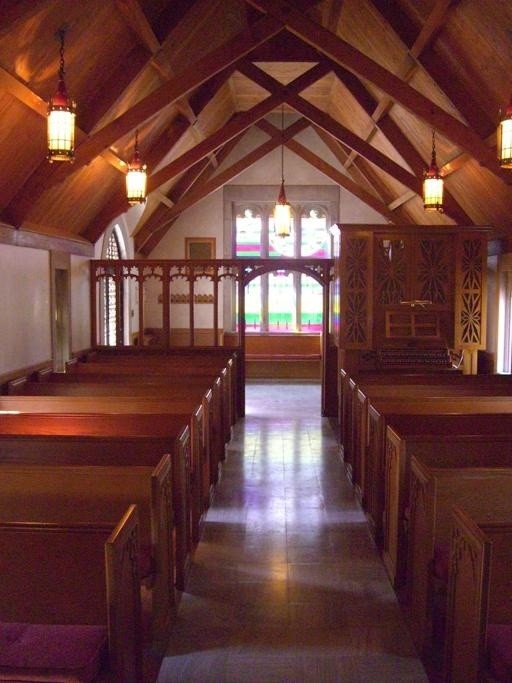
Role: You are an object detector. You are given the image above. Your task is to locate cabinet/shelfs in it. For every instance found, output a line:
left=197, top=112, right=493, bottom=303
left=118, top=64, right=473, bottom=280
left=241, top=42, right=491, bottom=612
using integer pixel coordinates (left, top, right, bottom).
left=329, top=221, right=488, bottom=351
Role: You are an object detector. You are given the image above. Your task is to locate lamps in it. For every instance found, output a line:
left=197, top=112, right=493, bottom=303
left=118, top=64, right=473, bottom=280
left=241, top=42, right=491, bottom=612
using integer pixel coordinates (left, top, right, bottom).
left=43, top=3, right=80, bottom=164
left=421, top=103, right=447, bottom=211
left=272, top=101, right=291, bottom=239
left=124, top=124, right=149, bottom=209
left=496, top=114, right=512, bottom=174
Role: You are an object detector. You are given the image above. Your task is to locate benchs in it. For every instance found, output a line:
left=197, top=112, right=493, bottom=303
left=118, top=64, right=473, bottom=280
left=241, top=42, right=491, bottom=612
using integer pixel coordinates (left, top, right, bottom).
left=0, top=342, right=249, bottom=680
left=329, top=347, right=512, bottom=682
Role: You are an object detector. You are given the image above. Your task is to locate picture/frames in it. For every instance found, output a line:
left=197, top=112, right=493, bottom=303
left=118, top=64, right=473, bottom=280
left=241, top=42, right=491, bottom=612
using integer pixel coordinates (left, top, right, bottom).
left=185, top=237, right=216, bottom=277
